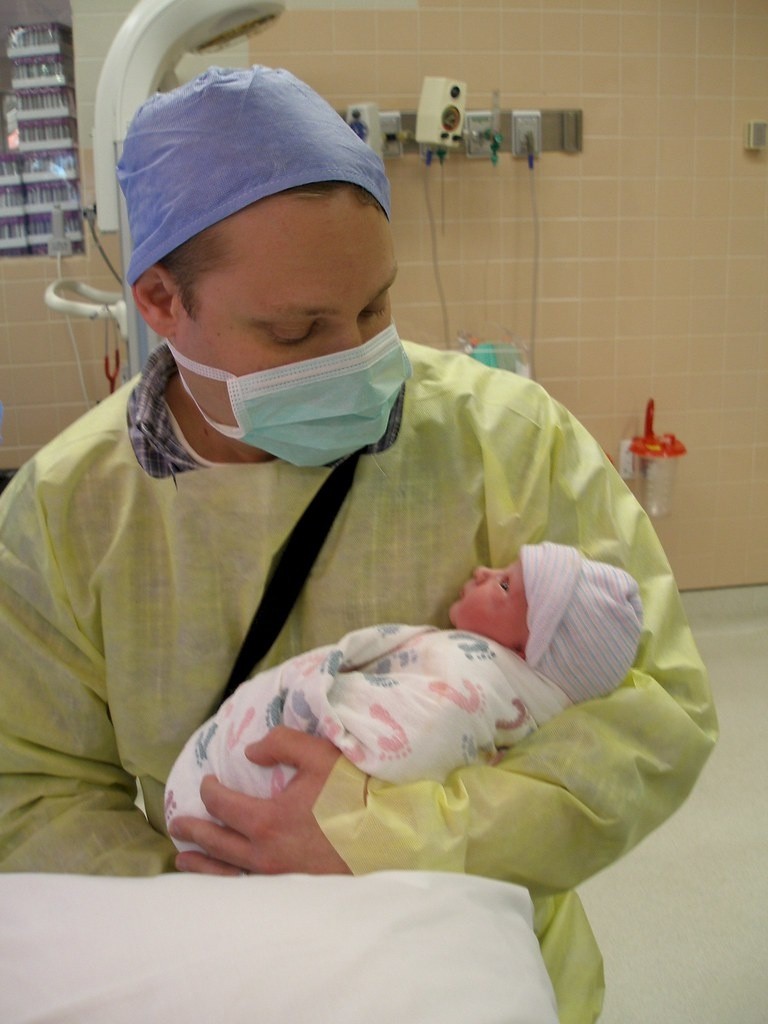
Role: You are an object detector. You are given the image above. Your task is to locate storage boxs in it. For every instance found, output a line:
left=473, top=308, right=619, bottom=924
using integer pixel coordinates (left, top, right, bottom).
left=0, top=21, right=83, bottom=256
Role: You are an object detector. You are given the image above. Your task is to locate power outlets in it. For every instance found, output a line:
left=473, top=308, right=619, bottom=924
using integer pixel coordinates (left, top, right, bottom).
left=464, top=110, right=495, bottom=159
left=510, top=109, right=543, bottom=159
left=378, top=111, right=405, bottom=161
left=420, top=143, right=450, bottom=160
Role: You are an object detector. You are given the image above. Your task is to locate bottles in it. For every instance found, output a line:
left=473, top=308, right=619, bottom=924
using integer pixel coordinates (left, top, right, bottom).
left=0, top=22, right=80, bottom=256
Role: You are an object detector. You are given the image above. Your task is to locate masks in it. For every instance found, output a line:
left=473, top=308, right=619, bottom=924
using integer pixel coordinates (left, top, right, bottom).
left=159, top=319, right=413, bottom=467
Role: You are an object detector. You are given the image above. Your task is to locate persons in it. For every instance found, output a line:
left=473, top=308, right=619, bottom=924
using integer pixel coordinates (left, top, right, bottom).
left=0, top=61, right=720, bottom=1024
left=165, top=541, right=643, bottom=860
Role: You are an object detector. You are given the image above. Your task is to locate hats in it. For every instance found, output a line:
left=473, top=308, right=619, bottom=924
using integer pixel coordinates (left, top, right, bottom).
left=114, top=64, right=392, bottom=290
left=520, top=540, right=646, bottom=705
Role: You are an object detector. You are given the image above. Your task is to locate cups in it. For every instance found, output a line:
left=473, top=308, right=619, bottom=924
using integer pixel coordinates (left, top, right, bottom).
left=630, top=399, right=688, bottom=517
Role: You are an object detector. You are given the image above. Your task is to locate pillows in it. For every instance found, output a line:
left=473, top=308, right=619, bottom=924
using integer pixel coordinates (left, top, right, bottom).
left=0, top=869, right=560, bottom=1024
